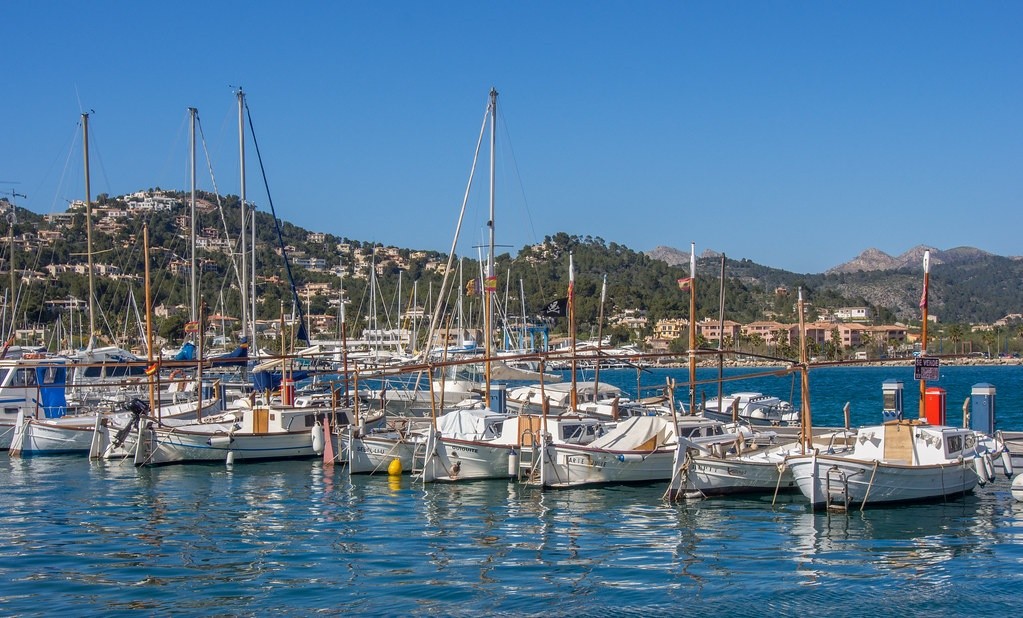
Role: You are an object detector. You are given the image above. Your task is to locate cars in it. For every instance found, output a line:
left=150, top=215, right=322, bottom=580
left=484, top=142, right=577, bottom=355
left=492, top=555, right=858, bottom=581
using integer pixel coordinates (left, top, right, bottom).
left=981, top=352, right=993, bottom=359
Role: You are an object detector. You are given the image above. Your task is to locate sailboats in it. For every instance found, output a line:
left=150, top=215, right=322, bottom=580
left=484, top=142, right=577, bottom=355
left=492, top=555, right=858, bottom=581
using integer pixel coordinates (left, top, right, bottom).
left=0, top=87, right=685, bottom=484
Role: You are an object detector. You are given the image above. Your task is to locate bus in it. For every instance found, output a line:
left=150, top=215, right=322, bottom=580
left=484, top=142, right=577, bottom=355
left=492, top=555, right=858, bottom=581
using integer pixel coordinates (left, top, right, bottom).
left=912, top=342, right=936, bottom=358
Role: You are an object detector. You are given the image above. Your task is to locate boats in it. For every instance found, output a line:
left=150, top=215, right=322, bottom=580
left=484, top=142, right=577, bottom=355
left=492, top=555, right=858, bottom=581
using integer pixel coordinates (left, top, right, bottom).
left=540, top=243, right=779, bottom=488
left=701, top=391, right=800, bottom=427
left=663, top=286, right=857, bottom=504
left=785, top=250, right=1013, bottom=515
